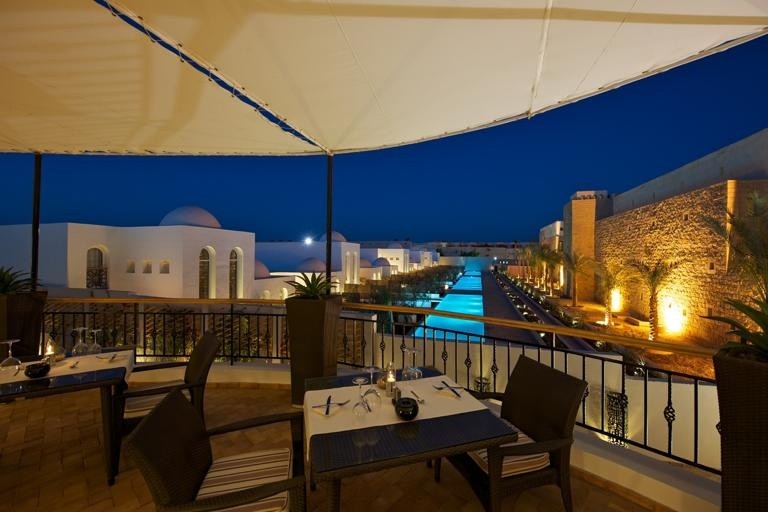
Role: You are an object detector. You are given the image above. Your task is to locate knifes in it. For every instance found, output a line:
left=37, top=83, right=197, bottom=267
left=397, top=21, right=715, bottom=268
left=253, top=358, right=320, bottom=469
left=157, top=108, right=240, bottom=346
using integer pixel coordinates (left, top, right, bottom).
left=441, top=381, right=461, bottom=398
left=325, top=395, right=331, bottom=415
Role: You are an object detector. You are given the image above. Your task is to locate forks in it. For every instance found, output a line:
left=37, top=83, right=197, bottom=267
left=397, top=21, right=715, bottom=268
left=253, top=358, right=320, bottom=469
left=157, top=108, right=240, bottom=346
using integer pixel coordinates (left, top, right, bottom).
left=312, top=400, right=350, bottom=407
left=432, top=384, right=465, bottom=390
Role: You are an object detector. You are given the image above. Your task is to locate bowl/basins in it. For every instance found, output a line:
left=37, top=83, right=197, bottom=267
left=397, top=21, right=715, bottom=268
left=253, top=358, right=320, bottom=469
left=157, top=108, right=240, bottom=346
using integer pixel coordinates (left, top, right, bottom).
left=25, top=363, right=50, bottom=378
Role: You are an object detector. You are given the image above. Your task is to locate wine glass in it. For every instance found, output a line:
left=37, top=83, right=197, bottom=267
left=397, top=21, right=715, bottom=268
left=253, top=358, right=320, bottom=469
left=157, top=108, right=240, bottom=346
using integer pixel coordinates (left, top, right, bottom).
left=0, top=340, right=23, bottom=378
left=352, top=365, right=382, bottom=417
left=402, top=347, right=423, bottom=385
left=71, top=327, right=102, bottom=358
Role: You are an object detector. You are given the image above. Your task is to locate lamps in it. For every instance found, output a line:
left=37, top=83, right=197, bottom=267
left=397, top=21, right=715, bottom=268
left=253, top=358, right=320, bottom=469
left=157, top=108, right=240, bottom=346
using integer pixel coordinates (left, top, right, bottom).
left=607, top=391, right=629, bottom=450
left=474, top=377, right=490, bottom=392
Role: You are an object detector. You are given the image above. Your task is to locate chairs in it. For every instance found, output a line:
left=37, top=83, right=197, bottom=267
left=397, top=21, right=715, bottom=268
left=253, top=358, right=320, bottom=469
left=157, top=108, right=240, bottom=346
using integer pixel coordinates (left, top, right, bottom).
left=434, top=355, right=588, bottom=512
left=123, top=388, right=307, bottom=512
left=111, top=331, right=223, bottom=476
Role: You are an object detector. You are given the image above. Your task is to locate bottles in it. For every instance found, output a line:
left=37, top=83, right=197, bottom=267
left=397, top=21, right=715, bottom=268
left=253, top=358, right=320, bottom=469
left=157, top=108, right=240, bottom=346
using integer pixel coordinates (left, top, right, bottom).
left=395, top=397, right=419, bottom=421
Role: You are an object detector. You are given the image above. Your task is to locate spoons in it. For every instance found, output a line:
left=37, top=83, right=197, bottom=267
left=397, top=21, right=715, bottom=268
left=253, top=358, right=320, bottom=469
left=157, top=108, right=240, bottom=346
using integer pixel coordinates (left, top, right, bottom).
left=410, top=391, right=425, bottom=404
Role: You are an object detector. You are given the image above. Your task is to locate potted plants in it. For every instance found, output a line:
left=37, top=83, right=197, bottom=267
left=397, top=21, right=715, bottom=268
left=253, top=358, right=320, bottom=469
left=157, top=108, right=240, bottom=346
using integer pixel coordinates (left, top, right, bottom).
left=697, top=290, right=768, bottom=512
left=285, top=270, right=342, bottom=405
left=0, top=264, right=48, bottom=359
